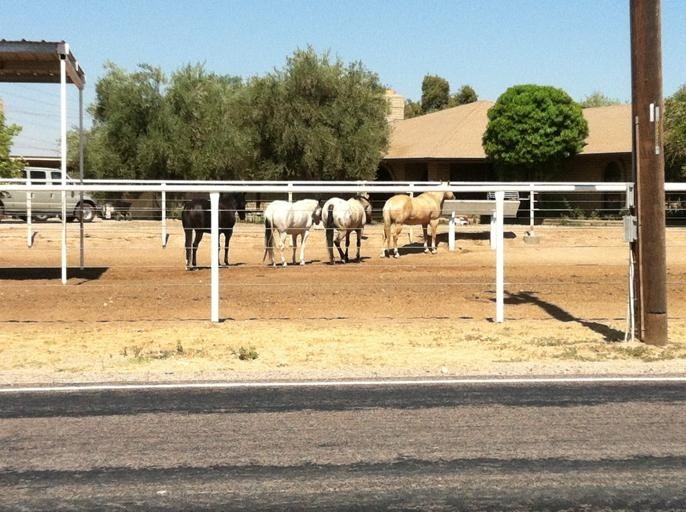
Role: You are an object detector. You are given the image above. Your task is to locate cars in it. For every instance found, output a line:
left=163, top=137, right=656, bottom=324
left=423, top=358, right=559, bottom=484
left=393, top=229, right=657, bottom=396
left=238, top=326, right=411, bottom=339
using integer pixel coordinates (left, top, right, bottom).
left=0, top=165, right=98, bottom=223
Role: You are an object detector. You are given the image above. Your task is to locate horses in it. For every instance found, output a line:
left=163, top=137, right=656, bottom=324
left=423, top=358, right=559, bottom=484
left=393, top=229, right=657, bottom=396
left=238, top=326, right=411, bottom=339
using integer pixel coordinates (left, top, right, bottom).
left=379, top=179, right=456, bottom=259
left=262, top=198, right=322, bottom=268
left=321, top=195, right=373, bottom=265
left=181, top=191, right=247, bottom=270
